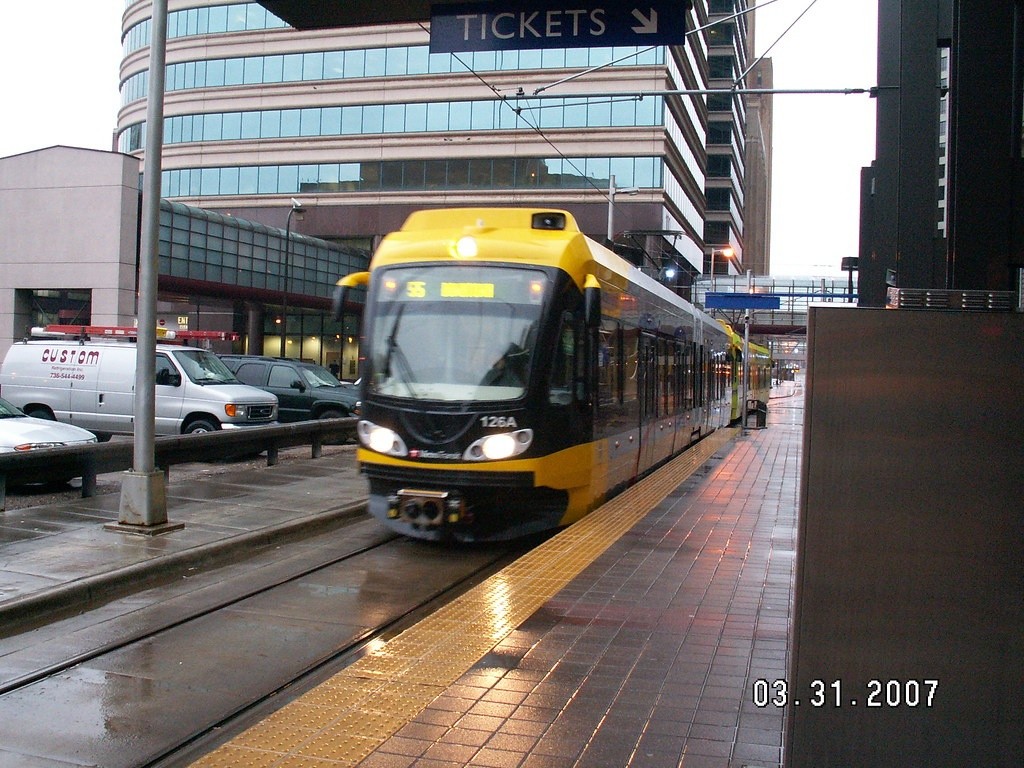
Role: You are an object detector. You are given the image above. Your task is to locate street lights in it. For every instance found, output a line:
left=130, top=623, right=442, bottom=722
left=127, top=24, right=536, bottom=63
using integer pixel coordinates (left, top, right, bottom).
left=606, top=174, right=641, bottom=252
left=278, top=205, right=307, bottom=360
left=708, top=248, right=734, bottom=316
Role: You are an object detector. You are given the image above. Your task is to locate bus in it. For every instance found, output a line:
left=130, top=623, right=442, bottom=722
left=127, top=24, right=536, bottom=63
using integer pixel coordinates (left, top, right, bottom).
left=330, top=206, right=774, bottom=546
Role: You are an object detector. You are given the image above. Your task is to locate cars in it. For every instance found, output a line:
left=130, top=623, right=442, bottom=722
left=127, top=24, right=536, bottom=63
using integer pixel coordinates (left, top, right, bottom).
left=0, top=397, right=99, bottom=485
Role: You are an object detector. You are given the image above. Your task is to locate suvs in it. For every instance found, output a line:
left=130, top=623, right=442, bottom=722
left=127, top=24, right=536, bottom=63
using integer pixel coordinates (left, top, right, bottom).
left=215, top=352, right=361, bottom=444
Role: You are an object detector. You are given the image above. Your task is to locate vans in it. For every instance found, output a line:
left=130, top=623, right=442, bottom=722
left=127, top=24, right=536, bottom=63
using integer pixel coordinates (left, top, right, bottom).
left=1, top=323, right=281, bottom=462
